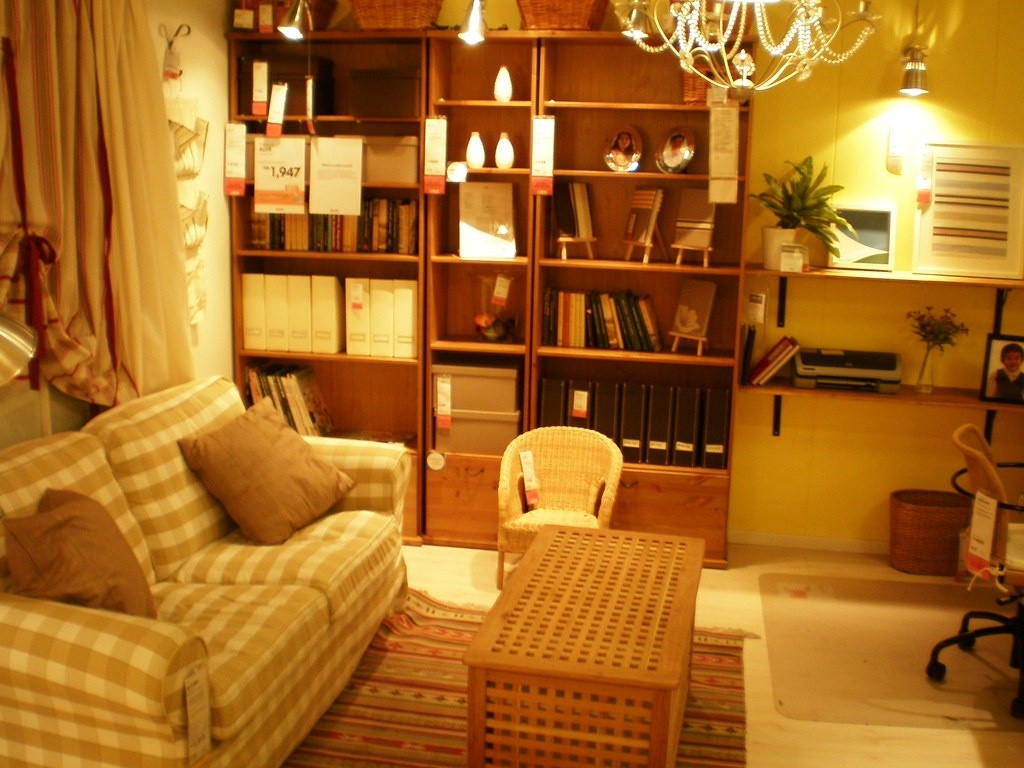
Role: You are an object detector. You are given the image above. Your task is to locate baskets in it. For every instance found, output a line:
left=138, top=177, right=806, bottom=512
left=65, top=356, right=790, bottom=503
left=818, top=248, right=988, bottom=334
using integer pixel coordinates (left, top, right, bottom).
left=349, top=0, right=444, bottom=31
left=683, top=64, right=741, bottom=105
left=516, top=0, right=610, bottom=31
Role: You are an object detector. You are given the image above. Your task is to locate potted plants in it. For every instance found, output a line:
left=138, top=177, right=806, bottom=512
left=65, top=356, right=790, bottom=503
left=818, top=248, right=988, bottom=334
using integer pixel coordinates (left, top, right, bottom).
left=749, top=156, right=858, bottom=270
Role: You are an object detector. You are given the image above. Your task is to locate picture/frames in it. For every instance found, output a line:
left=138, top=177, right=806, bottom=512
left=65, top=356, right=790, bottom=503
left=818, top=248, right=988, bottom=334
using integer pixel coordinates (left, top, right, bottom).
left=603, top=124, right=643, bottom=172
left=979, top=333, right=1024, bottom=405
left=911, top=137, right=1024, bottom=280
left=653, top=127, right=695, bottom=174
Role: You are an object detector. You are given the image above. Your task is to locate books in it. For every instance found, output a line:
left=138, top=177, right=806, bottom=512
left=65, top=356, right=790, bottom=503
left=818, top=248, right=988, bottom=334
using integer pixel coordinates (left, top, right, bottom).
left=247, top=194, right=417, bottom=255
left=457, top=180, right=517, bottom=259
left=543, top=288, right=662, bottom=352
left=247, top=360, right=333, bottom=439
left=751, top=336, right=800, bottom=387
left=563, top=180, right=716, bottom=250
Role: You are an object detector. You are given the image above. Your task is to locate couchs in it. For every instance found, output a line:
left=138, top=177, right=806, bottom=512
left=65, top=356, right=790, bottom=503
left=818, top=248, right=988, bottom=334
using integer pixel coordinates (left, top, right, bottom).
left=0, top=375, right=412, bottom=768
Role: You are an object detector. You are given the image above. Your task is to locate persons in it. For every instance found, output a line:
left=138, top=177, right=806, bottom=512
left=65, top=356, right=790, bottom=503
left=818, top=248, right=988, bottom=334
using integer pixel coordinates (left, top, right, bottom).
left=987, top=343, right=1024, bottom=404
left=663, top=135, right=689, bottom=168
left=612, top=132, right=633, bottom=167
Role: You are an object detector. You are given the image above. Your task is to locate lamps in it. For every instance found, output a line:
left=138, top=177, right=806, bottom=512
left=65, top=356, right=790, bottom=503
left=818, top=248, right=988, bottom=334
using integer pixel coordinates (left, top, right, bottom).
left=618, top=0, right=654, bottom=39
left=276, top=0, right=313, bottom=40
left=899, top=0, right=930, bottom=97
left=0, top=310, right=38, bottom=389
left=609, top=0, right=883, bottom=101
left=458, top=0, right=488, bottom=45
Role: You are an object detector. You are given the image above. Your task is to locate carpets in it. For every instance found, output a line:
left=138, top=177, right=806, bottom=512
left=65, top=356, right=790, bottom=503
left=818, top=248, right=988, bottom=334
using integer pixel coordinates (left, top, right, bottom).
left=277, top=587, right=762, bottom=768
left=759, top=572, right=1024, bottom=731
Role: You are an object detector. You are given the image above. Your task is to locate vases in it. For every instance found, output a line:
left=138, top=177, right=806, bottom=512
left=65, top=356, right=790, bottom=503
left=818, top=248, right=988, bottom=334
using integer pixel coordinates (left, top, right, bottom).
left=915, top=344, right=934, bottom=393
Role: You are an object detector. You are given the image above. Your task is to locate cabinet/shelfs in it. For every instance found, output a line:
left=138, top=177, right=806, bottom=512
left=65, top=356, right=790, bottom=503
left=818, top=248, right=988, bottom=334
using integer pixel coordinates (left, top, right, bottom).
left=739, top=261, right=1024, bottom=446
left=227, top=32, right=758, bottom=571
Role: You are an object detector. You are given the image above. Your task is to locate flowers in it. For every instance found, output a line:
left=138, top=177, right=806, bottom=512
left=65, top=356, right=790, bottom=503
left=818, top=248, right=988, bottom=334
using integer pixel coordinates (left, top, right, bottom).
left=905, top=307, right=969, bottom=356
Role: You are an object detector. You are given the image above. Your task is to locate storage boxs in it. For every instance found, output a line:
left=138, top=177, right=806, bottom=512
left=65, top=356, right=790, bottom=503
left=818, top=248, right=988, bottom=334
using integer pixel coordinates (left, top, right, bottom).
left=345, top=278, right=370, bottom=356
left=540, top=378, right=567, bottom=428
left=432, top=364, right=518, bottom=412
left=644, top=383, right=674, bottom=465
left=394, top=280, right=417, bottom=358
left=245, top=133, right=309, bottom=180
left=594, top=382, right=618, bottom=442
left=671, top=385, right=699, bottom=468
left=566, top=379, right=593, bottom=429
left=334, top=135, right=419, bottom=184
left=700, top=387, right=731, bottom=469
left=618, top=381, right=646, bottom=463
left=311, top=275, right=345, bottom=354
left=435, top=408, right=520, bottom=457
left=265, top=274, right=288, bottom=352
left=242, top=273, right=266, bottom=350
left=287, top=275, right=311, bottom=353
left=369, top=278, right=394, bottom=358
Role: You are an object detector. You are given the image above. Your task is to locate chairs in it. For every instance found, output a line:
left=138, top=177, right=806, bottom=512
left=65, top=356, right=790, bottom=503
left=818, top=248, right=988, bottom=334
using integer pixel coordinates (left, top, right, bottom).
left=924, top=424, right=1024, bottom=722
left=497, top=427, right=624, bottom=589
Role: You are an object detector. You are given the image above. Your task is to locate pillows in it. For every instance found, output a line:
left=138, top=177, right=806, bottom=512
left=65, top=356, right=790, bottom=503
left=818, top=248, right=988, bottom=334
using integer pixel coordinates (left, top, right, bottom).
left=2, top=487, right=158, bottom=619
left=177, top=395, right=357, bottom=544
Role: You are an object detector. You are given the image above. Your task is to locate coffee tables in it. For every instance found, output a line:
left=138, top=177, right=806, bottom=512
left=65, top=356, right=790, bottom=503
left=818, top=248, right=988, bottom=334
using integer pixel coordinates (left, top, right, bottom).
left=461, top=524, right=705, bottom=768
